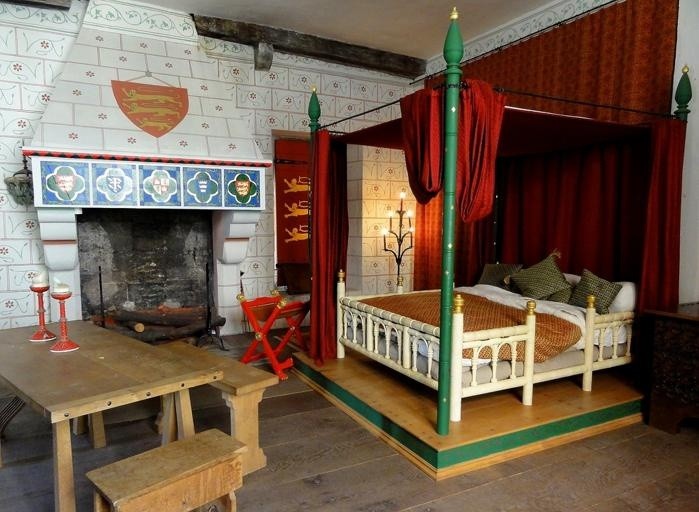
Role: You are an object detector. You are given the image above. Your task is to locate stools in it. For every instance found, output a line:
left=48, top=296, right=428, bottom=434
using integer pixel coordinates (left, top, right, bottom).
left=157, top=340, right=278, bottom=477
left=87, top=429, right=248, bottom=512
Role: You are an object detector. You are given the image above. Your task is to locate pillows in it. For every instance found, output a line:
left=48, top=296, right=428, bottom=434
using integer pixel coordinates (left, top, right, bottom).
left=568, top=269, right=622, bottom=314
left=563, top=274, right=636, bottom=311
left=478, top=263, right=522, bottom=291
left=509, top=249, right=571, bottom=300
left=539, top=274, right=572, bottom=304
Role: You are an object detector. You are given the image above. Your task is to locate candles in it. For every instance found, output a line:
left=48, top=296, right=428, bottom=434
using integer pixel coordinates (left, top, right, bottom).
left=54, top=283, right=70, bottom=292
left=32, top=278, right=48, bottom=287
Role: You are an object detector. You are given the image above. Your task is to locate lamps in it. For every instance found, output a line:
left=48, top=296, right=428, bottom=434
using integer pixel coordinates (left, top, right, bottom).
left=381, top=191, right=415, bottom=285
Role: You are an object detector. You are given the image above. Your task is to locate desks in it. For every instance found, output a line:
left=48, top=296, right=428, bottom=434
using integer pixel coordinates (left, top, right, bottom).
left=0, top=320, right=223, bottom=512
left=336, top=268, right=635, bottom=422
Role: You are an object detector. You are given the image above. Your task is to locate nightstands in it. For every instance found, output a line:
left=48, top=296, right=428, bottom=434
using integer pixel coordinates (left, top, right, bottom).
left=644, top=302, right=699, bottom=435
